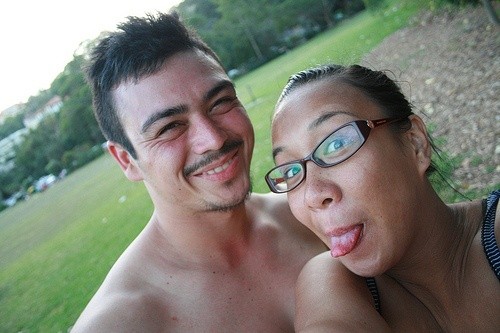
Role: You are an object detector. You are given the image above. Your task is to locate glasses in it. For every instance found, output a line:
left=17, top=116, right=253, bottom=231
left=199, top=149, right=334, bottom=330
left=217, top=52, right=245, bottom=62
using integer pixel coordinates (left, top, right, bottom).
left=264, top=111, right=415, bottom=195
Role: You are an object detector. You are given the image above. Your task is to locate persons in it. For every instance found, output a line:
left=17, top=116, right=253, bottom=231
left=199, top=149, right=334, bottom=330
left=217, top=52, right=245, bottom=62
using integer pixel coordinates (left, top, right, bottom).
left=265, top=63, right=499, bottom=333
left=69, top=11, right=332, bottom=333
